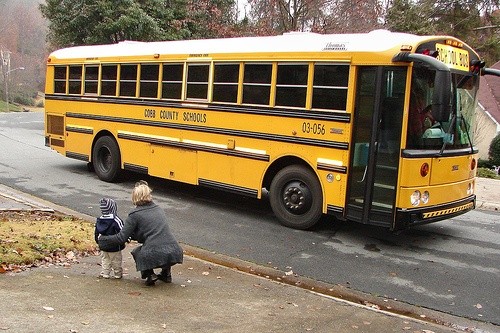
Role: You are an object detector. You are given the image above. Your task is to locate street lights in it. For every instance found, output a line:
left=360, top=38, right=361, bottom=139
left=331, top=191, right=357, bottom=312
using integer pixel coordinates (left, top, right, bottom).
left=5, top=67, right=25, bottom=113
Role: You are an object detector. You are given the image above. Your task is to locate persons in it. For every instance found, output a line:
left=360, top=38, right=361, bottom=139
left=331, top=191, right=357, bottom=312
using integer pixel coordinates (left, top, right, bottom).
left=94, top=197, right=131, bottom=279
left=409, top=88, right=437, bottom=150
left=98, top=184, right=183, bottom=285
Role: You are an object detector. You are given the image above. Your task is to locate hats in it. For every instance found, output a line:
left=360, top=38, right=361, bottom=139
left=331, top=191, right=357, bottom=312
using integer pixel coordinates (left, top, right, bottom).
left=99, top=197, right=117, bottom=215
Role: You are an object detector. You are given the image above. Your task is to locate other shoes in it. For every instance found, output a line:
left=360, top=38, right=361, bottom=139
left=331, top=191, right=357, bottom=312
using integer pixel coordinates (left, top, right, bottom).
left=145, top=273, right=158, bottom=286
left=114, top=275, right=122, bottom=279
left=101, top=271, right=110, bottom=278
left=157, top=272, right=172, bottom=283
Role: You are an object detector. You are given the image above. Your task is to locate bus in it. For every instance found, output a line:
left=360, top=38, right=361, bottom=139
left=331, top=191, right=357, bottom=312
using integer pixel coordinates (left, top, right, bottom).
left=43, top=29, right=500, bottom=231
